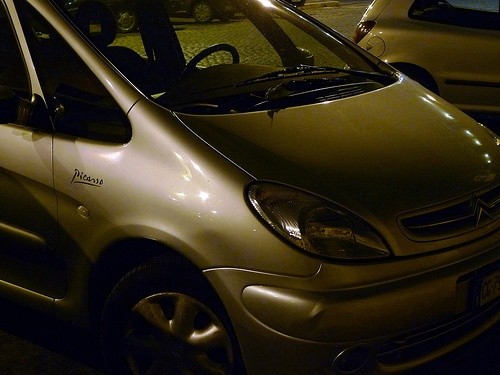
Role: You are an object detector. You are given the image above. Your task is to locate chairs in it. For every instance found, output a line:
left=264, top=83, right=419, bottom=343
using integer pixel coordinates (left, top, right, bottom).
left=93, top=46, right=154, bottom=101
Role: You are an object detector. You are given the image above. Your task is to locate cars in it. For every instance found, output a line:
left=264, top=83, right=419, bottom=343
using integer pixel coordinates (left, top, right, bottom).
left=338, top=0, right=500, bottom=120
left=0, top=0, right=500, bottom=375
left=109, top=1, right=239, bottom=34
